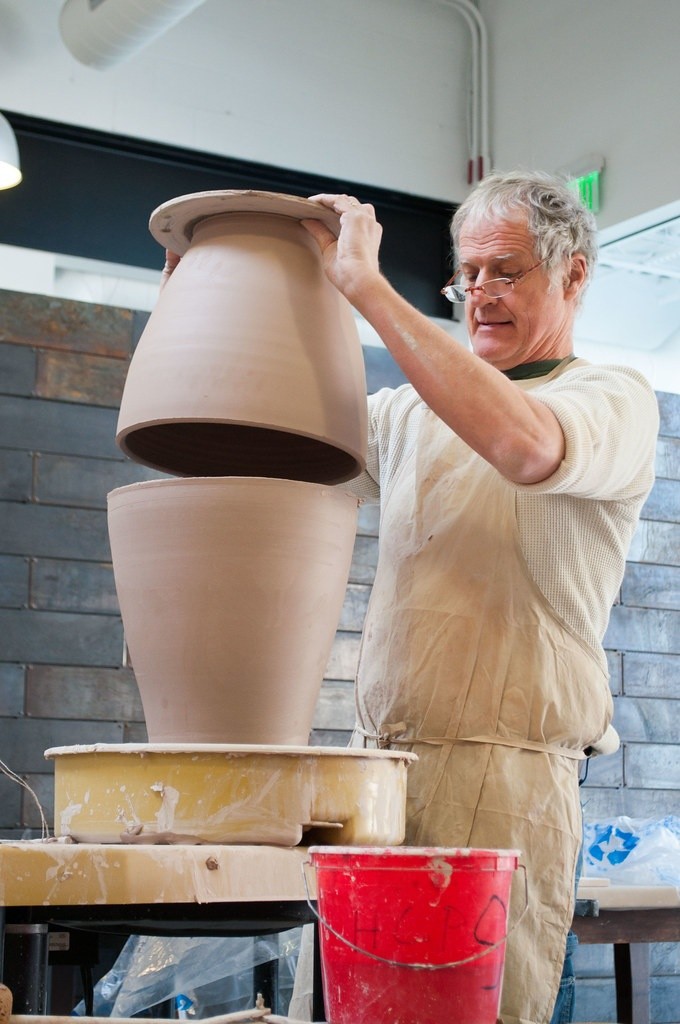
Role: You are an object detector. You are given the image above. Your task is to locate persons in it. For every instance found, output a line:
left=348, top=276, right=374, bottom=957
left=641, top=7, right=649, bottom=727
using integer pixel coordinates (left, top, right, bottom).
left=156, top=171, right=659, bottom=1024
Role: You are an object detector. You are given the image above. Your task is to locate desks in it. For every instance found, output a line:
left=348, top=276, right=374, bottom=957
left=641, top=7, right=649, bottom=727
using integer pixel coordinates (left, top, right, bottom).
left=0, top=841, right=327, bottom=1024
left=571, top=886, right=680, bottom=1024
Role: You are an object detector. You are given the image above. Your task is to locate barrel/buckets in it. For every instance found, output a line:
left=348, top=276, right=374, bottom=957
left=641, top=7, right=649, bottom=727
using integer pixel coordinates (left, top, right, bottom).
left=300, top=846, right=529, bottom=1024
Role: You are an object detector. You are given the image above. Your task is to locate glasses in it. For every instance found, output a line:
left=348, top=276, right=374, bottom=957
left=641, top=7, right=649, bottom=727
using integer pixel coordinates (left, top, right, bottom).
left=441, top=254, right=550, bottom=303
